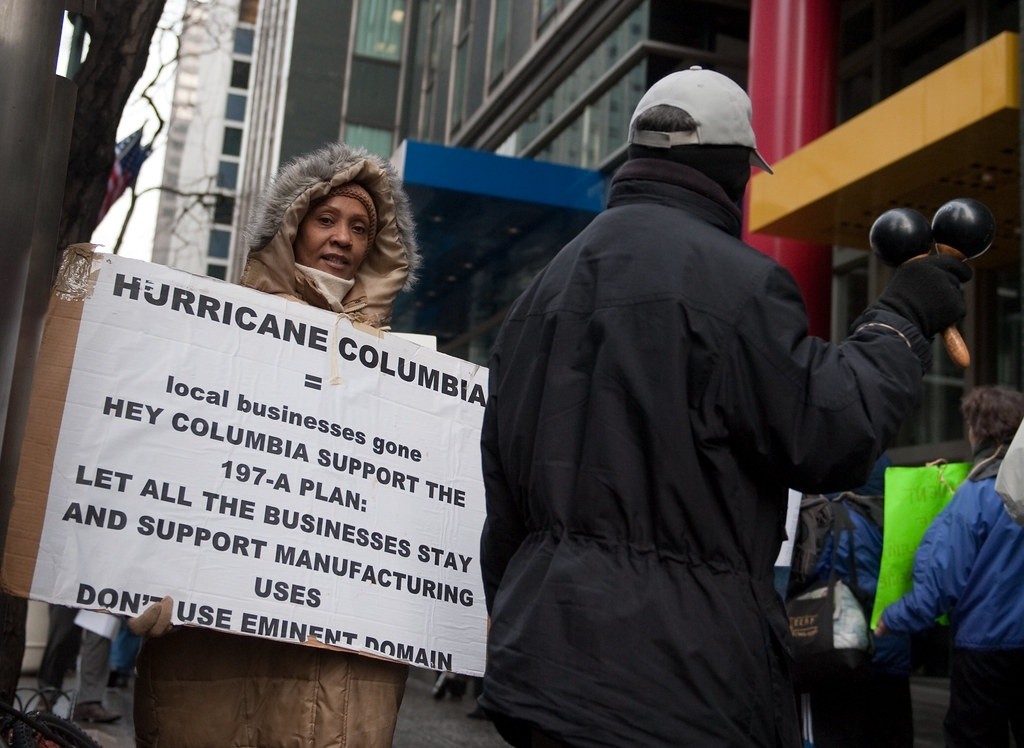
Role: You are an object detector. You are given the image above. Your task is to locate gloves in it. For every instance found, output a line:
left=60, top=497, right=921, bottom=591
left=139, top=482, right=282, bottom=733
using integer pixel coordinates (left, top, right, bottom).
left=864, top=254, right=972, bottom=345
left=127, top=595, right=173, bottom=636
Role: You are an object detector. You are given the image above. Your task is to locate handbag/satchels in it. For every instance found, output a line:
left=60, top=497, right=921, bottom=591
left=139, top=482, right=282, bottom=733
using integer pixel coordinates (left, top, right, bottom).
left=785, top=501, right=872, bottom=678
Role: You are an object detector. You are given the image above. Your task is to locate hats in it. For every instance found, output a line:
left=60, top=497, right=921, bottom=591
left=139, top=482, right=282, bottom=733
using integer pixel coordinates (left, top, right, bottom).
left=318, top=182, right=377, bottom=256
left=628, top=66, right=774, bottom=174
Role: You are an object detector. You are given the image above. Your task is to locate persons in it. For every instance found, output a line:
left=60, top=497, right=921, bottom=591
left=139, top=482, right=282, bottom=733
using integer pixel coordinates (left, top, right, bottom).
left=433, top=671, right=490, bottom=720
left=480, top=65, right=973, bottom=748
left=875, top=383, right=1024, bottom=748
left=128, top=144, right=422, bottom=748
left=995, top=418, right=1024, bottom=525
left=807, top=453, right=908, bottom=748
left=34, top=604, right=139, bottom=722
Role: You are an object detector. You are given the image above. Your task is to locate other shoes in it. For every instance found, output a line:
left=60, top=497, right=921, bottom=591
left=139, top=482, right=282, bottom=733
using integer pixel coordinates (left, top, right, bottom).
left=35, top=699, right=53, bottom=713
left=105, top=669, right=125, bottom=692
left=72, top=700, right=122, bottom=724
left=466, top=705, right=489, bottom=720
